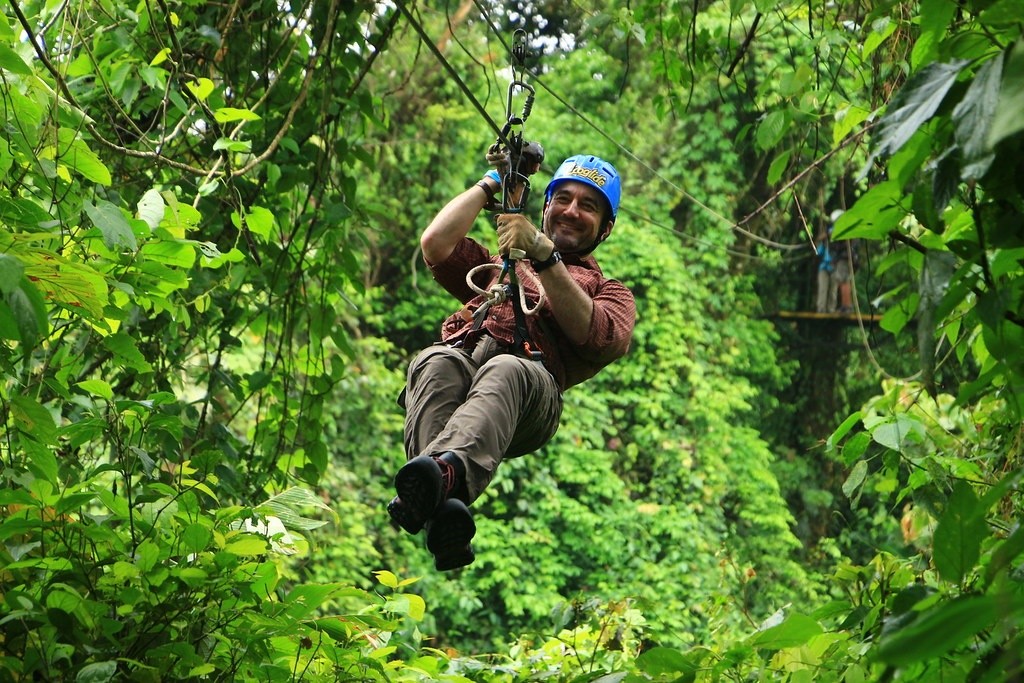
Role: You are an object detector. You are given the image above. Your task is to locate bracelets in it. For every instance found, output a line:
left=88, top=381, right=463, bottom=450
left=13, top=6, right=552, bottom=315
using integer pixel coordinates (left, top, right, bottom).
left=476, top=179, right=494, bottom=210
left=484, top=170, right=501, bottom=186
left=529, top=251, right=562, bottom=273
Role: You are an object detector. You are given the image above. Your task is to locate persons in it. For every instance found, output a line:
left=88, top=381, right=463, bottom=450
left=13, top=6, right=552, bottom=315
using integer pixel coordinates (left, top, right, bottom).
left=813, top=229, right=860, bottom=314
left=387, top=141, right=638, bottom=572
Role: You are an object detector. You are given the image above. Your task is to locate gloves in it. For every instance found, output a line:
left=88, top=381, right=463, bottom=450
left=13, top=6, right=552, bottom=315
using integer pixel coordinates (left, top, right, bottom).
left=494, top=214, right=555, bottom=262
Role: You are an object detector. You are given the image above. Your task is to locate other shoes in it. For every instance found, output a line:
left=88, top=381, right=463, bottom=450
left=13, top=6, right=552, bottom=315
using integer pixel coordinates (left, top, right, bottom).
left=428, top=498, right=476, bottom=571
left=387, top=452, right=465, bottom=536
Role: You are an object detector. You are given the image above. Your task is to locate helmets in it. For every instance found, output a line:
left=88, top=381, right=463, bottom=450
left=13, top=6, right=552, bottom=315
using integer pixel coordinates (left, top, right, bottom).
left=545, top=154, right=621, bottom=224
